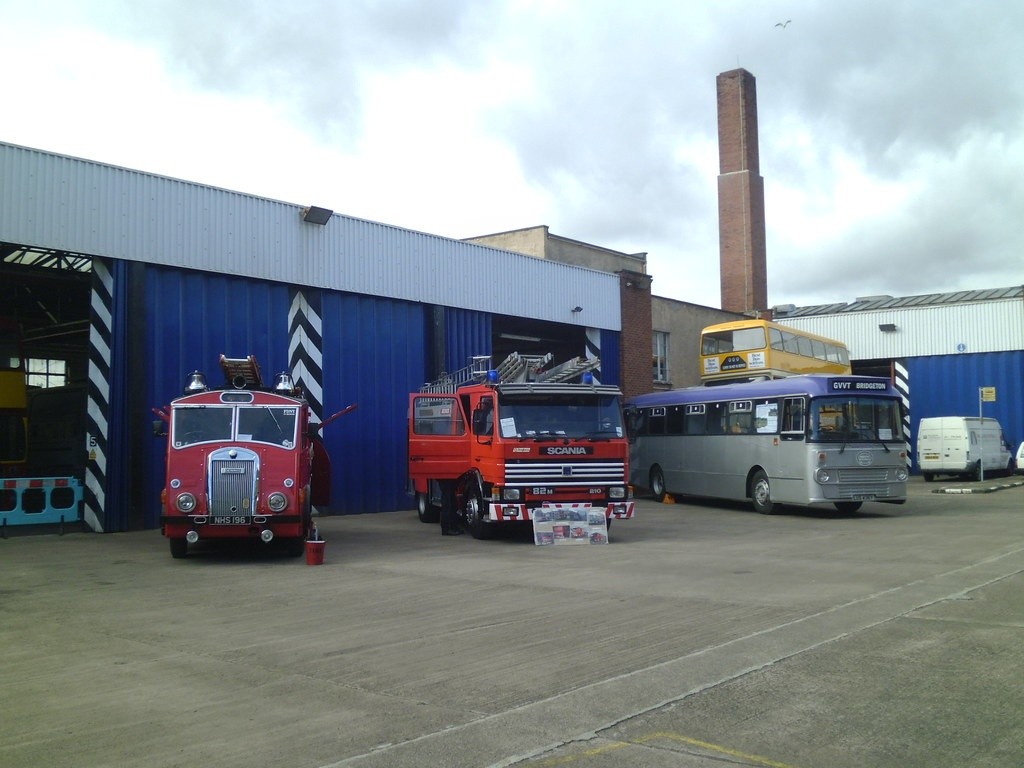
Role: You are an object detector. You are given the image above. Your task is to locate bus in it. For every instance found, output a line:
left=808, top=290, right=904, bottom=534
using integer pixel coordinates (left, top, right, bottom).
left=624, top=374, right=909, bottom=515
left=699, top=319, right=852, bottom=433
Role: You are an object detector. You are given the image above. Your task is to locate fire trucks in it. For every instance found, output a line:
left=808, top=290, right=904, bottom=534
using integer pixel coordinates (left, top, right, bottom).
left=153, top=354, right=360, bottom=559
left=406, top=350, right=635, bottom=540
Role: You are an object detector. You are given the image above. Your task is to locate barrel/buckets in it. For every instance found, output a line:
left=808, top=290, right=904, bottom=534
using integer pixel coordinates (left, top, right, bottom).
left=304, top=540, right=327, bottom=565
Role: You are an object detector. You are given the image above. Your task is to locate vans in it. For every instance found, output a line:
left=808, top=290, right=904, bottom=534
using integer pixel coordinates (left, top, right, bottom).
left=916, top=416, right=1014, bottom=483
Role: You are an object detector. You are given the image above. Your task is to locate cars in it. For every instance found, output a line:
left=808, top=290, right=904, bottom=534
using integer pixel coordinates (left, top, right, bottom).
left=1014, top=442, right=1024, bottom=478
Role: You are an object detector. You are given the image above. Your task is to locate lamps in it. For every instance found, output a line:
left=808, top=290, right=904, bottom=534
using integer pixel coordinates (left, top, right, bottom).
left=298, top=206, right=334, bottom=226
left=879, top=323, right=897, bottom=332
